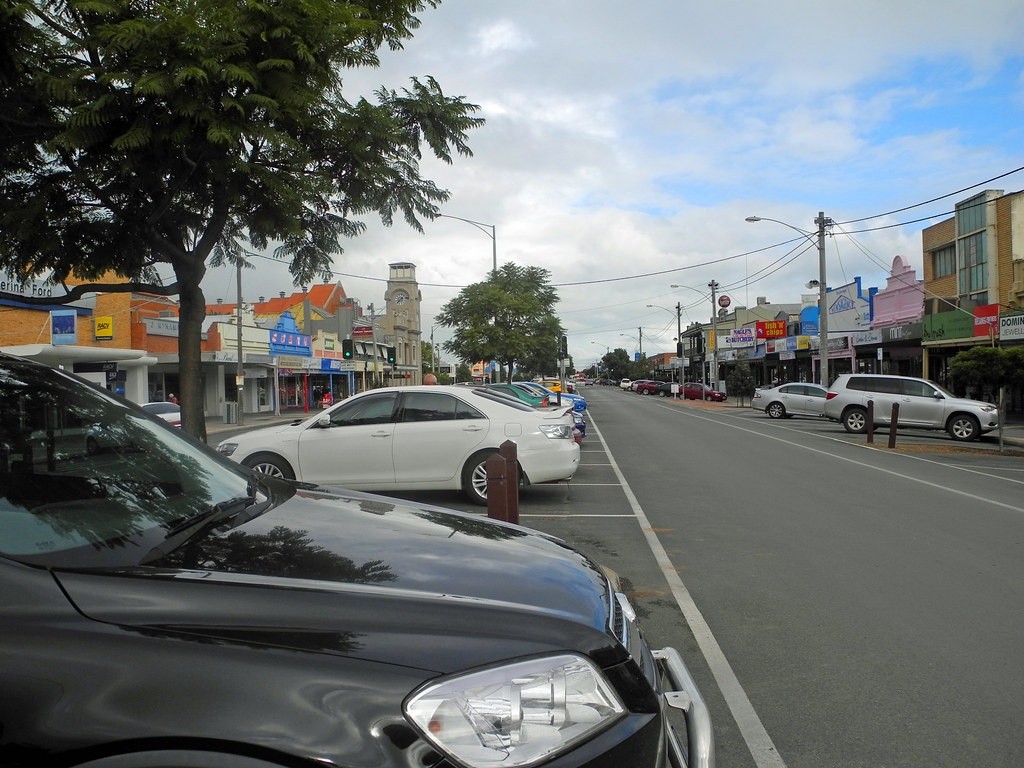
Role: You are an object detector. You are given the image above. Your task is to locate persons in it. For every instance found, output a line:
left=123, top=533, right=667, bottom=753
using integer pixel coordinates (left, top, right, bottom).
left=168, top=393, right=177, bottom=403
left=423, top=373, right=438, bottom=385
left=315, top=387, right=328, bottom=407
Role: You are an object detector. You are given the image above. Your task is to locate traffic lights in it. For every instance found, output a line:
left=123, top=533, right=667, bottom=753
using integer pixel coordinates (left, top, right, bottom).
left=342, top=339, right=353, bottom=359
left=387, top=348, right=396, bottom=363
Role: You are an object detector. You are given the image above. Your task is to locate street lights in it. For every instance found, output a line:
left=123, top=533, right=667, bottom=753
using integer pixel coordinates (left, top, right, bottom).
left=670, top=285, right=718, bottom=391
left=591, top=341, right=610, bottom=387
left=434, top=213, right=501, bottom=383
left=745, top=217, right=829, bottom=389
left=646, top=304, right=684, bottom=400
left=620, top=334, right=641, bottom=353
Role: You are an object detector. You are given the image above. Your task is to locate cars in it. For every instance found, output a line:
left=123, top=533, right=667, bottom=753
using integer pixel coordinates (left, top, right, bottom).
left=485, top=378, right=588, bottom=438
left=85, top=401, right=181, bottom=454
left=753, top=383, right=830, bottom=419
left=585, top=379, right=593, bottom=386
left=824, top=373, right=999, bottom=442
left=654, top=382, right=682, bottom=397
left=215, top=386, right=580, bottom=506
left=0, top=351, right=718, bottom=768
left=594, top=378, right=617, bottom=385
left=676, top=382, right=727, bottom=402
left=620, top=379, right=651, bottom=394
left=637, top=381, right=663, bottom=395
